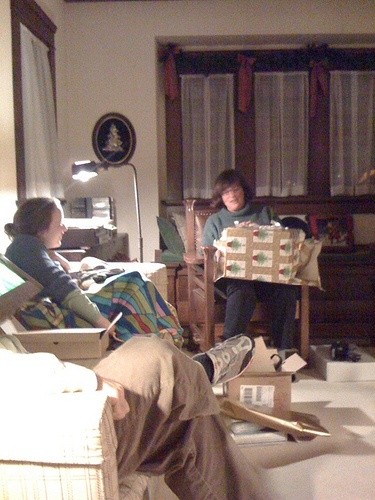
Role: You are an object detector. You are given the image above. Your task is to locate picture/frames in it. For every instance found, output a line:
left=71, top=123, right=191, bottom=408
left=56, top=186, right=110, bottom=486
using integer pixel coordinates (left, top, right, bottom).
left=309, top=214, right=355, bottom=253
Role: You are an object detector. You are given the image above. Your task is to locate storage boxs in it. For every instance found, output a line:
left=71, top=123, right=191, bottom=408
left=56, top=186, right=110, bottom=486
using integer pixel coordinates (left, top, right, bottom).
left=0, top=312, right=123, bottom=359
left=314, top=344, right=375, bottom=382
left=212, top=227, right=324, bottom=293
left=219, top=336, right=308, bottom=446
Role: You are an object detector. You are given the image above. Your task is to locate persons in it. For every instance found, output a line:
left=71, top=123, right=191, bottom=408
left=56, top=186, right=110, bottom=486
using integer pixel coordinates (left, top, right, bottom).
left=4, top=197, right=184, bottom=350
left=0, top=328, right=271, bottom=500
left=198, top=169, right=296, bottom=383
left=328, top=223, right=341, bottom=244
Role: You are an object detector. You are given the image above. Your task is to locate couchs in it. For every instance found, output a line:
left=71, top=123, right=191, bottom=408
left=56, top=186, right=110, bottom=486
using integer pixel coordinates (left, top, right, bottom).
left=153, top=196, right=375, bottom=344
left=0, top=257, right=167, bottom=500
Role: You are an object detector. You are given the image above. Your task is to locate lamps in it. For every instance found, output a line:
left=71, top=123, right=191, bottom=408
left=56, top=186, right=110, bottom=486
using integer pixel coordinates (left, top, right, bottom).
left=72, top=159, right=144, bottom=263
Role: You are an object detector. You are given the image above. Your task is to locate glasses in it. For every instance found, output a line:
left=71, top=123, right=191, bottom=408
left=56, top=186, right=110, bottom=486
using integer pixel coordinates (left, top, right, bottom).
left=222, top=187, right=243, bottom=195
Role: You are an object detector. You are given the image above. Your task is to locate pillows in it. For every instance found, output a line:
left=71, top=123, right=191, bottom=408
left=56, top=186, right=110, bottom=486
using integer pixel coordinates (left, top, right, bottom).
left=173, top=213, right=185, bottom=246
left=155, top=216, right=184, bottom=255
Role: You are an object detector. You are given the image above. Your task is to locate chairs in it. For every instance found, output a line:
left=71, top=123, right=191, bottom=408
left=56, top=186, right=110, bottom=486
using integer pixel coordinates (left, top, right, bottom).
left=183, top=198, right=310, bottom=360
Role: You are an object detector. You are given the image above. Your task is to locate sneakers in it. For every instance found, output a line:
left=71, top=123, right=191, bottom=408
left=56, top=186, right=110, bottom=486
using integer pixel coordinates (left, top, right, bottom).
left=191, top=333, right=256, bottom=387
